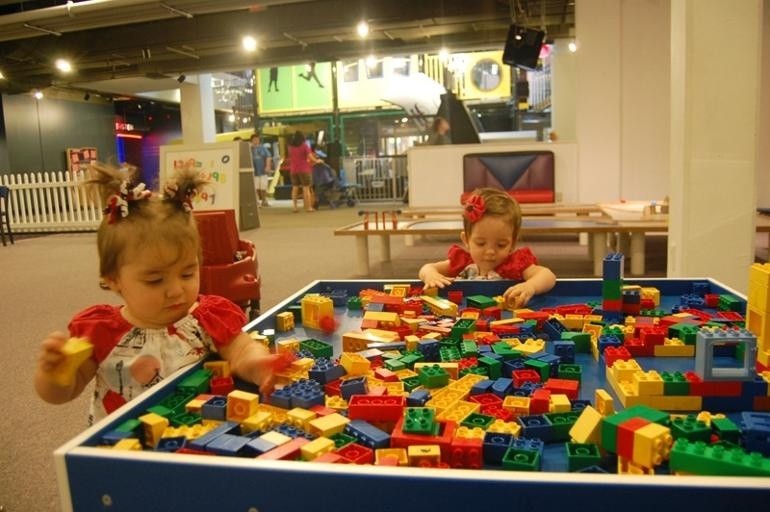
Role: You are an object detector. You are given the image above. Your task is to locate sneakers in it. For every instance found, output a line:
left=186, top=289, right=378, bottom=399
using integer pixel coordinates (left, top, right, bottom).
left=261, top=202, right=272, bottom=207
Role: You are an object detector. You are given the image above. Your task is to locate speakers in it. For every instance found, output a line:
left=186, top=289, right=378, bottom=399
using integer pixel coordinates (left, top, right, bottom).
left=501, top=22, right=546, bottom=72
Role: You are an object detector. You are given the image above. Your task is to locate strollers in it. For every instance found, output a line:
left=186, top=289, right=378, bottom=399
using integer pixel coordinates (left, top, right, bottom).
left=311, top=162, right=357, bottom=209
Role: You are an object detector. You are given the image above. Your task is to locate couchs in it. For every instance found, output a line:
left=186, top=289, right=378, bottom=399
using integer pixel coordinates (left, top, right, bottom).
left=459, top=150, right=555, bottom=216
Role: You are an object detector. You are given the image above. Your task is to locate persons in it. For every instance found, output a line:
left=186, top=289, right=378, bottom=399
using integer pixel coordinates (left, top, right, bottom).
left=297, top=63, right=325, bottom=89
left=267, top=67, right=280, bottom=94
left=418, top=187, right=557, bottom=310
left=31, top=160, right=305, bottom=430
left=249, top=128, right=340, bottom=213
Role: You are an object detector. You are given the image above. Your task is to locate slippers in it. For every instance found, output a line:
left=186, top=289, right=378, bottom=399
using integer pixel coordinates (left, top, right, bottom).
left=293, top=209, right=316, bottom=212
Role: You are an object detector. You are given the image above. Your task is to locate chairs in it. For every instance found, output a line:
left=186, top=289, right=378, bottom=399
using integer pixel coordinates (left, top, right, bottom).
left=0, top=186, right=14, bottom=246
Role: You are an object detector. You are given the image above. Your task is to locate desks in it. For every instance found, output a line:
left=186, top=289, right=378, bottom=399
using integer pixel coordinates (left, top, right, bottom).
left=52, top=278, right=769, bottom=511
